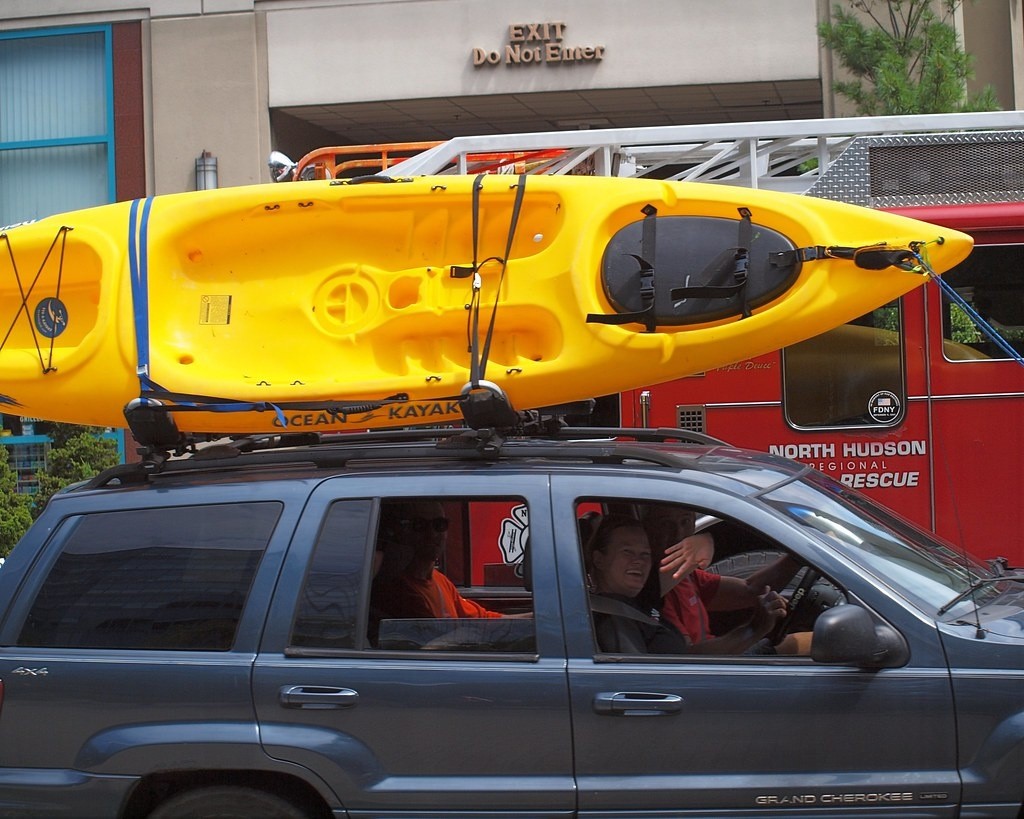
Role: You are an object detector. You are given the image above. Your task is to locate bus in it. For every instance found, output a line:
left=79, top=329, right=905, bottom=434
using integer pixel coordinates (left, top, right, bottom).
left=164, top=109, right=1024, bottom=573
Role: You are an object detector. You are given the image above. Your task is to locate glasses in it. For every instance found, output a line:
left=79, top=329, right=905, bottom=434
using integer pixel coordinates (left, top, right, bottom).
left=391, top=516, right=447, bottom=532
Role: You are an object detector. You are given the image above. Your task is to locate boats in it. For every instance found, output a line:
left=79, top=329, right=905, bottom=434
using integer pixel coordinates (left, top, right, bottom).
left=0, top=174, right=974, bottom=432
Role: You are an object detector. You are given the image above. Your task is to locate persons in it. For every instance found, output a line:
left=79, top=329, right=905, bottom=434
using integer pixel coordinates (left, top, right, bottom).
left=586, top=515, right=733, bottom=658
left=644, top=501, right=816, bottom=657
left=365, top=496, right=534, bottom=652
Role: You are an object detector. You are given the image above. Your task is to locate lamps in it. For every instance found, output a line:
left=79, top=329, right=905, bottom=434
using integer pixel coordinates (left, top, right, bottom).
left=195, top=150, right=219, bottom=191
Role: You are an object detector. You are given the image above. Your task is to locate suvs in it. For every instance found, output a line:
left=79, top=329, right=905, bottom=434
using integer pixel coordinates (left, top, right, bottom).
left=0, top=426, right=1024, bottom=819
